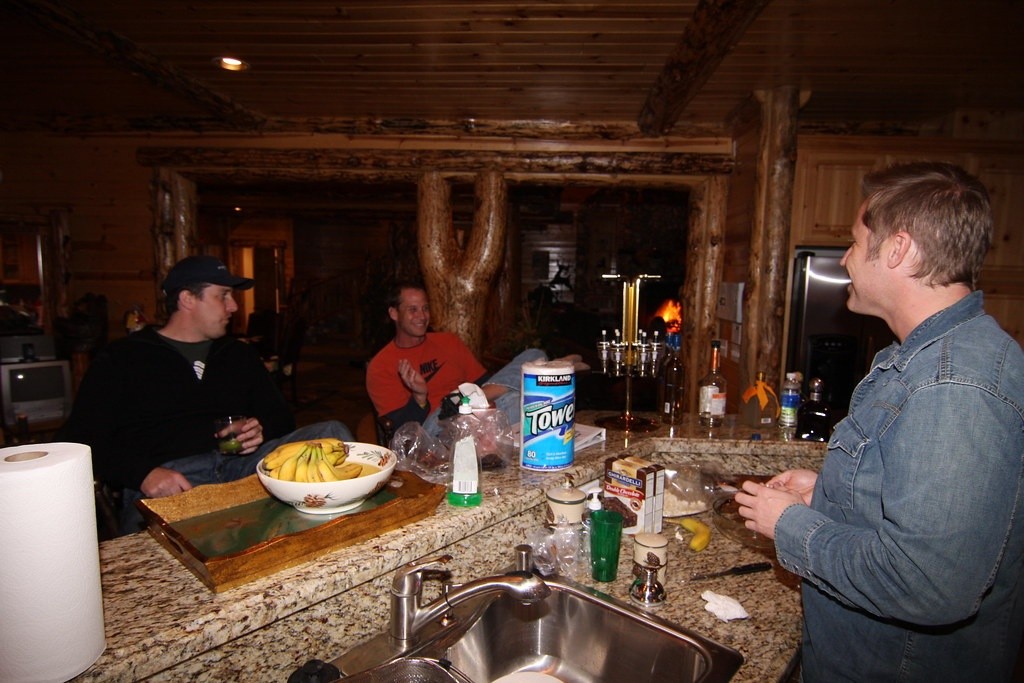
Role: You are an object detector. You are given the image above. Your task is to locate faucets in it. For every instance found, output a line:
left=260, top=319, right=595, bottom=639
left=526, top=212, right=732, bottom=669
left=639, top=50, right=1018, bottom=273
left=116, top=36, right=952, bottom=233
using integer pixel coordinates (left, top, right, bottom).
left=389, top=551, right=552, bottom=649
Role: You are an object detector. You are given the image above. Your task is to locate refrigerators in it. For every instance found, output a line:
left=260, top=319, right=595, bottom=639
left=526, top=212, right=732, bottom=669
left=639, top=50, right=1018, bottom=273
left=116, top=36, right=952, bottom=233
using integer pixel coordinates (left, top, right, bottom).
left=785, top=249, right=901, bottom=443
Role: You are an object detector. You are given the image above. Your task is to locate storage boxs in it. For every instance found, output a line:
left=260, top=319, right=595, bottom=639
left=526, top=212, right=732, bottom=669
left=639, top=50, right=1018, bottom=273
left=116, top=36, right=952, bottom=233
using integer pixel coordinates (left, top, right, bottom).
left=605, top=455, right=665, bottom=536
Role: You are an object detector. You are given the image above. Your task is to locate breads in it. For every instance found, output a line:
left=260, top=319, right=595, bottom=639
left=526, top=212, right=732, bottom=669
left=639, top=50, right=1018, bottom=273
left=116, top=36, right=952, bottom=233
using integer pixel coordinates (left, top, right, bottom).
left=601, top=497, right=637, bottom=527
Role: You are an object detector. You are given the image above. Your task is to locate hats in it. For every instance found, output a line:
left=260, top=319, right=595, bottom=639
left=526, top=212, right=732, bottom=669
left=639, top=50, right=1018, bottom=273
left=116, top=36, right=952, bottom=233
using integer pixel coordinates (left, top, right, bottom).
left=161, top=255, right=255, bottom=298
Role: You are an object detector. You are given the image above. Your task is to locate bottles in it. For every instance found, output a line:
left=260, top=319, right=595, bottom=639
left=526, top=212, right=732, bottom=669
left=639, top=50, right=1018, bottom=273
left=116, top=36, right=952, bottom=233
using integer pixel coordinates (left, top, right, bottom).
left=447, top=395, right=482, bottom=509
left=696, top=340, right=727, bottom=428
left=658, top=331, right=685, bottom=424
left=736, top=370, right=777, bottom=429
left=596, top=326, right=664, bottom=364
left=795, top=378, right=831, bottom=443
left=777, top=372, right=802, bottom=429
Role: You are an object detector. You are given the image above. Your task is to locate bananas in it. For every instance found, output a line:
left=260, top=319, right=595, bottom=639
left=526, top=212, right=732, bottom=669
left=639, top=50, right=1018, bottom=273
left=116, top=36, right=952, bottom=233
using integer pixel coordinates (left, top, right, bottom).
left=262, top=438, right=362, bottom=483
left=666, top=516, right=711, bottom=551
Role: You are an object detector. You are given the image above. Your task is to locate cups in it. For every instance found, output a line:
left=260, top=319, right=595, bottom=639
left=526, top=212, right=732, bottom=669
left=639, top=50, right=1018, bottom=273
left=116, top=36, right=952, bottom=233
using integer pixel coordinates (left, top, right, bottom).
left=590, top=509, right=625, bottom=583
left=215, top=414, right=247, bottom=455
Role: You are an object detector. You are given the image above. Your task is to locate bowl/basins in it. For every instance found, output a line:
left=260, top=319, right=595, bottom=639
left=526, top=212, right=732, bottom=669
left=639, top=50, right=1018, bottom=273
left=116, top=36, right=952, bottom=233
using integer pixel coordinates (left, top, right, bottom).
left=712, top=495, right=775, bottom=548
left=256, top=442, right=397, bottom=516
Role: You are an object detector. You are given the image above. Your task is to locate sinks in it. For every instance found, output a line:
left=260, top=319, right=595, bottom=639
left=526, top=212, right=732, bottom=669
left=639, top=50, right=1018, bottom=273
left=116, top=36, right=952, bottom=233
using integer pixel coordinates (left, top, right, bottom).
left=414, top=581, right=711, bottom=683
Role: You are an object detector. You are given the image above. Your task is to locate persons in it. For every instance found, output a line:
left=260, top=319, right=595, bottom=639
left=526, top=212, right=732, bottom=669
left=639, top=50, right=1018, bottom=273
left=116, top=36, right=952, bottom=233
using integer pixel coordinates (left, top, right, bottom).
left=365, top=282, right=591, bottom=445
left=54, top=256, right=357, bottom=535
left=735, top=161, right=1024, bottom=683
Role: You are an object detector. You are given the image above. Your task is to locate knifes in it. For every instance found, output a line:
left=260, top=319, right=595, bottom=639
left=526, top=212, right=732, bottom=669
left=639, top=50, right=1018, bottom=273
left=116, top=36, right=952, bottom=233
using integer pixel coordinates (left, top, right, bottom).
left=689, top=561, right=772, bottom=582
